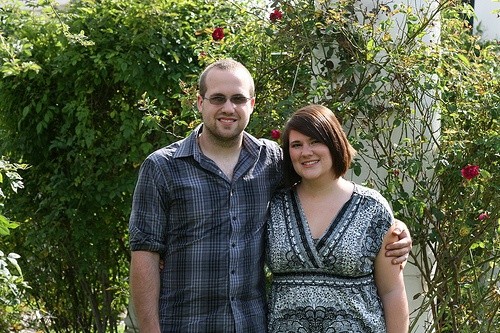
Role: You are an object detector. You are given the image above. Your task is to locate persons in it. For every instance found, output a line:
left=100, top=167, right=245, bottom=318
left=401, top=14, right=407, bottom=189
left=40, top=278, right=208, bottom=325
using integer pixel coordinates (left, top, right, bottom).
left=128, top=59, right=412, bottom=333
left=159, top=105, right=409, bottom=333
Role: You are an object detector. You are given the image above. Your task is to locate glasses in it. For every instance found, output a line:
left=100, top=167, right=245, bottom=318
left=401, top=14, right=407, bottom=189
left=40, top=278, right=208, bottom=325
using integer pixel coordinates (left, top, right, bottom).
left=204, top=93, right=253, bottom=105
